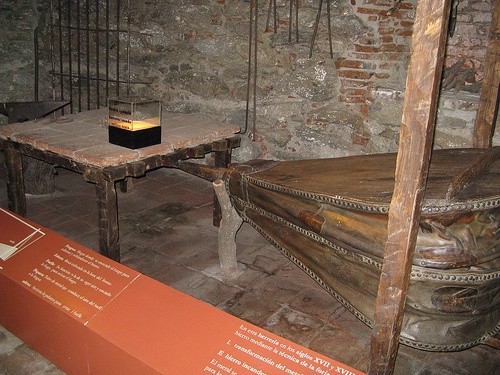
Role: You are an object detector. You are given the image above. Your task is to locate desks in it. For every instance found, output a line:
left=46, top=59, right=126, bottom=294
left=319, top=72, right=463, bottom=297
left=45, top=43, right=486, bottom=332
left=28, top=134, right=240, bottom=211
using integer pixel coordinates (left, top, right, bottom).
left=0, top=102, right=240, bottom=272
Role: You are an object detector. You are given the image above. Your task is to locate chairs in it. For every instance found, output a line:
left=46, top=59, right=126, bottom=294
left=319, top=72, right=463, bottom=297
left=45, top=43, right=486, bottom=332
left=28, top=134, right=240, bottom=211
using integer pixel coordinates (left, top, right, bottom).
left=223, top=0, right=499, bottom=375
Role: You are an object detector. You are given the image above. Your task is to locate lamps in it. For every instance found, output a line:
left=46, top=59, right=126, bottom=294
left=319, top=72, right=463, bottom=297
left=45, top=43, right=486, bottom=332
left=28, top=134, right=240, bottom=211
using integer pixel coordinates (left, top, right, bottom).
left=108, top=95, right=163, bottom=149
